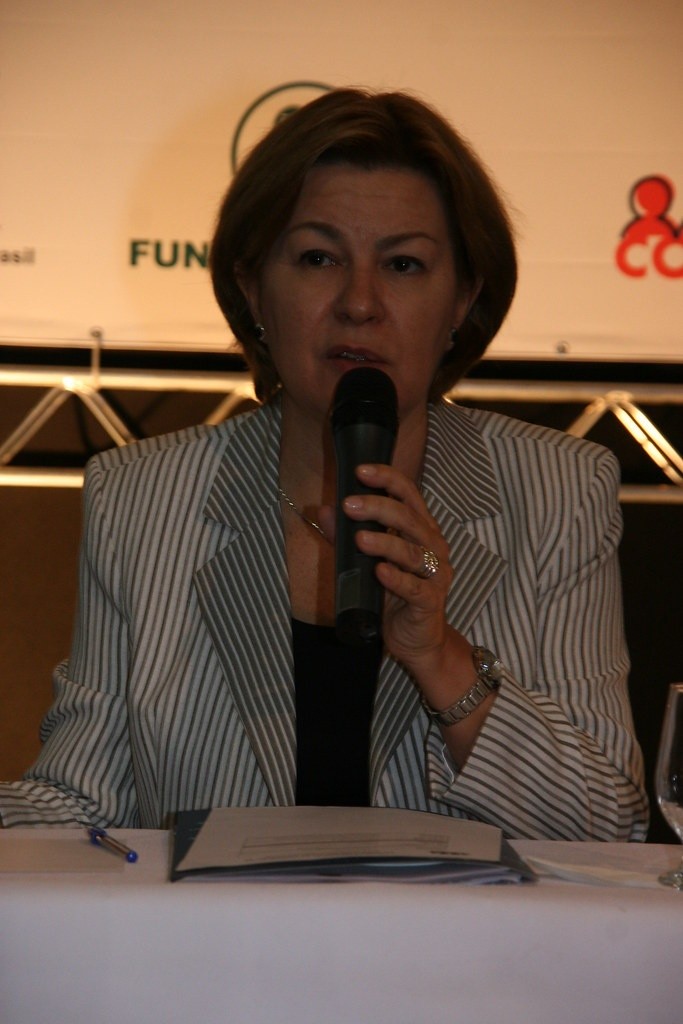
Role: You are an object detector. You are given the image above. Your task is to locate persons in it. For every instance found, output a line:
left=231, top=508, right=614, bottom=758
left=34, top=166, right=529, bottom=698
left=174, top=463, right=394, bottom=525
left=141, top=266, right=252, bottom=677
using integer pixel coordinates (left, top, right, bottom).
left=0, top=89, right=648, bottom=844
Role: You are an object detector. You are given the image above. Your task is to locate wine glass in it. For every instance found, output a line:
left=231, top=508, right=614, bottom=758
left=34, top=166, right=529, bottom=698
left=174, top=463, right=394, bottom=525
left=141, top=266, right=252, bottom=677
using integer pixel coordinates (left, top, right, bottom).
left=655, top=685, right=683, bottom=890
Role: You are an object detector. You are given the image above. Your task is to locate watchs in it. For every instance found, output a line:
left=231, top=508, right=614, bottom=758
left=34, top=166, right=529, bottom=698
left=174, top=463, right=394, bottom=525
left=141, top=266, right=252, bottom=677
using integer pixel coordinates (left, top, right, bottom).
left=421, top=646, right=501, bottom=726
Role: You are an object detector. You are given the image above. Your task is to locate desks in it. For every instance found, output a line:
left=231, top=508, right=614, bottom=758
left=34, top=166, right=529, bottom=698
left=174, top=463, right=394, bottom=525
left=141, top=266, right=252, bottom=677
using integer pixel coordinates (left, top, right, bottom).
left=1, top=827, right=683, bottom=1024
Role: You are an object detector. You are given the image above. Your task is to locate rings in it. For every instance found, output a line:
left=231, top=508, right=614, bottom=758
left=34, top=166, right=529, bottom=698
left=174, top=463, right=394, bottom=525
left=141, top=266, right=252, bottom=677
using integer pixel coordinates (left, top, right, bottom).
left=421, top=547, right=438, bottom=578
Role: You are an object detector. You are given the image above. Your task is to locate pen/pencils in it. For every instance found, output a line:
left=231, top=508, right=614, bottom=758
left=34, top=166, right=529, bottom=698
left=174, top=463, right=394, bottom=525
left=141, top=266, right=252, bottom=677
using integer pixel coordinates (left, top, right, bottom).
left=76, top=817, right=140, bottom=865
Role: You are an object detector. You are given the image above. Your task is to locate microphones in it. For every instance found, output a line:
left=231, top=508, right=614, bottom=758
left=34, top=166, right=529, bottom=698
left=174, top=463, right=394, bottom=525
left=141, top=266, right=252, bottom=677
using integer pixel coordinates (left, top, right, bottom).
left=330, top=365, right=398, bottom=635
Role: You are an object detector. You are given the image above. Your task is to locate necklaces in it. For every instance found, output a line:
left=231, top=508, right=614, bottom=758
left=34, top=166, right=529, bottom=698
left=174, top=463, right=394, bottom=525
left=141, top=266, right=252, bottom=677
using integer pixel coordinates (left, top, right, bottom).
left=277, top=487, right=336, bottom=547
